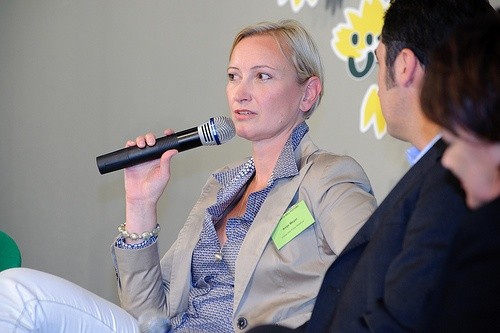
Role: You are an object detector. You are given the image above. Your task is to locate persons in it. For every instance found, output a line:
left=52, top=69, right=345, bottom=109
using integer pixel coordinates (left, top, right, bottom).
left=0, top=15, right=380, bottom=333
left=239, top=0, right=500, bottom=333
left=419, top=5, right=500, bottom=209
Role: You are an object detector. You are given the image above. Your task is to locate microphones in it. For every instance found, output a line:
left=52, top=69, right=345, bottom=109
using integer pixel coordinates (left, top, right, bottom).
left=95, top=114, right=236, bottom=178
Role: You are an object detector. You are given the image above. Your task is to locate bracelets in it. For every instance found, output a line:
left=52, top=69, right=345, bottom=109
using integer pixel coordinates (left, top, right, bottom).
left=117, top=223, right=161, bottom=241
left=115, top=235, right=159, bottom=250
